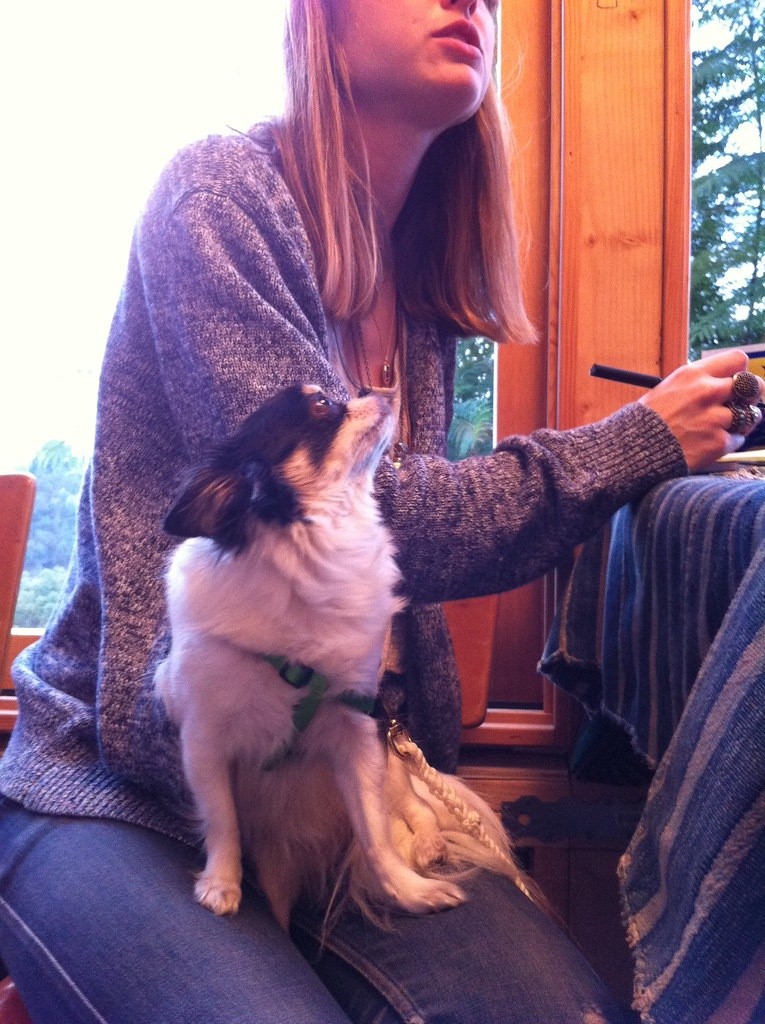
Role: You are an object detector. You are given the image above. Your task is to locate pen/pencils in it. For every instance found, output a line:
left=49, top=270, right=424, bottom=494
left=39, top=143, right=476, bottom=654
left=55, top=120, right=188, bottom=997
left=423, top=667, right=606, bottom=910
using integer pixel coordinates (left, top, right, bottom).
left=588, top=365, right=663, bottom=389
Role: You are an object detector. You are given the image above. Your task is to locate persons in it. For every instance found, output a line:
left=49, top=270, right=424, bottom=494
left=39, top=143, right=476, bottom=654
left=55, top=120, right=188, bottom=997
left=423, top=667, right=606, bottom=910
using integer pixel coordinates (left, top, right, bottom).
left=0, top=0, right=765, bottom=1024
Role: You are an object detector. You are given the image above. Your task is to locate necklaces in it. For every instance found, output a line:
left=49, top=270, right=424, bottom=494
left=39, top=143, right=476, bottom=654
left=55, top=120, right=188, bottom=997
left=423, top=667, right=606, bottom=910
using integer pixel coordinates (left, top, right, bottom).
left=371, top=283, right=396, bottom=384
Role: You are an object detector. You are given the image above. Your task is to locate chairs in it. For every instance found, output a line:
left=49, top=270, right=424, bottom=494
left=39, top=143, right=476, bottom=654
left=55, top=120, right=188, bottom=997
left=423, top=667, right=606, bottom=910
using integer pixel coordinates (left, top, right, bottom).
left=0, top=472, right=48, bottom=1024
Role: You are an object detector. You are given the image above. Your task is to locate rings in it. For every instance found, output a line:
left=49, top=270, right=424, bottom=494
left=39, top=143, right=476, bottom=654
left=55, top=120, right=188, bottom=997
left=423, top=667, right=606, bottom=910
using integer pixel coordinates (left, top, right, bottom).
left=726, top=402, right=758, bottom=436
left=732, top=371, right=761, bottom=402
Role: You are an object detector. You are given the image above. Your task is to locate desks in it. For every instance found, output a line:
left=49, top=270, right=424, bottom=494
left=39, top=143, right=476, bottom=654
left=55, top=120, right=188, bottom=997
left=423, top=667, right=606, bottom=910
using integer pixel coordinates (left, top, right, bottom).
left=536, top=472, right=765, bottom=1024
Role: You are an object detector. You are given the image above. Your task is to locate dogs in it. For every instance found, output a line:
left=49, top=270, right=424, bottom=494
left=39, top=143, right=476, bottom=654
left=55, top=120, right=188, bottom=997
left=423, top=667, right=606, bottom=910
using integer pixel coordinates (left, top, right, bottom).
left=156, top=384, right=518, bottom=917
